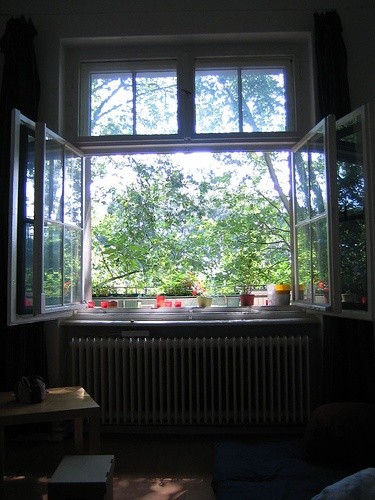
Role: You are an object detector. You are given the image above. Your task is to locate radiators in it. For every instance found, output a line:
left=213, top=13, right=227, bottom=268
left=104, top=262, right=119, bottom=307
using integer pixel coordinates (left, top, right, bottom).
left=69, top=335, right=313, bottom=442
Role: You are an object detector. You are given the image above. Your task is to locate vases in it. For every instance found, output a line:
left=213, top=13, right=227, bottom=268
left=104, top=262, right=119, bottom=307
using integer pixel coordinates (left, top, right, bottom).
left=196, top=297, right=214, bottom=307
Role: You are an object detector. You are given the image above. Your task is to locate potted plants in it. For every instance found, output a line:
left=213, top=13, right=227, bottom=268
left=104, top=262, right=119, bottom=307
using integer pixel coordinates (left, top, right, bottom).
left=239, top=289, right=255, bottom=306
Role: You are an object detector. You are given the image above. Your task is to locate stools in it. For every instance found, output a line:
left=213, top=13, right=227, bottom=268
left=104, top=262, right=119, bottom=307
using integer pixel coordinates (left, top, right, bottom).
left=46, top=454, right=117, bottom=500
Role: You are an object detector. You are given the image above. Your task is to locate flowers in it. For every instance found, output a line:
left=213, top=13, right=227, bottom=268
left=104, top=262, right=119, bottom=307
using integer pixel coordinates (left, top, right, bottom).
left=191, top=282, right=216, bottom=297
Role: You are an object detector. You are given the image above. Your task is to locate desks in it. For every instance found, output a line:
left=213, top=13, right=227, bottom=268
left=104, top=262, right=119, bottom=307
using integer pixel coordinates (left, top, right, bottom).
left=0, top=385, right=101, bottom=485
